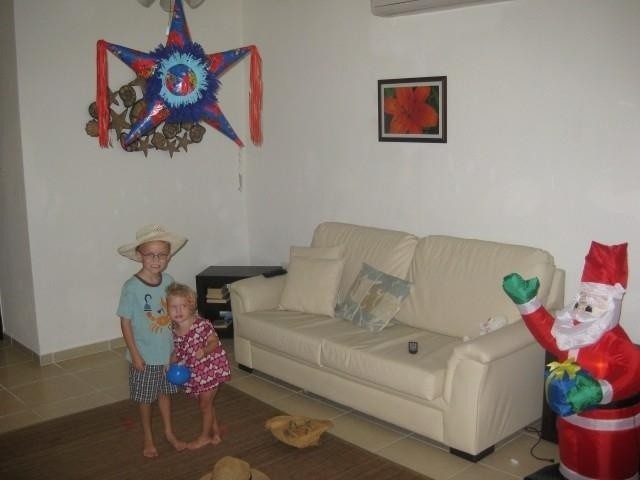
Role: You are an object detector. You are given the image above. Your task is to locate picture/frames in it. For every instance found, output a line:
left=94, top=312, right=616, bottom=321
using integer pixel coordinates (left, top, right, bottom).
left=377, top=75, right=449, bottom=146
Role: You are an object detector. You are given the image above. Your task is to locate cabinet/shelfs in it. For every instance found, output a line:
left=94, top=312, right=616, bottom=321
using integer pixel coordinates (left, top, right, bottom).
left=196, top=265, right=284, bottom=339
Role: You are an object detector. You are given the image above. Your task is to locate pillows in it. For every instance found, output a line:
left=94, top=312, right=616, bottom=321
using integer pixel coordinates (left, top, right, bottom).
left=280, top=244, right=417, bottom=335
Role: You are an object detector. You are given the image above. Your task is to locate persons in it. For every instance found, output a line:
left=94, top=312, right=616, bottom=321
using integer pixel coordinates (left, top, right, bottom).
left=497, top=238, right=640, bottom=480
left=165, top=282, right=231, bottom=451
left=117, top=222, right=186, bottom=459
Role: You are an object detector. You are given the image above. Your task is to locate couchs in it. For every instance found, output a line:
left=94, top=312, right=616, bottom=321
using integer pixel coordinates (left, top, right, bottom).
left=229, top=222, right=564, bottom=465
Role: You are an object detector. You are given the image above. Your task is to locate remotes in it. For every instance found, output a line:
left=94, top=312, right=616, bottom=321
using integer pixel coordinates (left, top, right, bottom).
left=408, top=341, right=418, bottom=353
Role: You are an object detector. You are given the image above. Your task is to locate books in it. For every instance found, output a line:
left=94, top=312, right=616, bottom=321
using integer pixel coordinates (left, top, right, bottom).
left=205, top=286, right=230, bottom=304
left=211, top=318, right=233, bottom=330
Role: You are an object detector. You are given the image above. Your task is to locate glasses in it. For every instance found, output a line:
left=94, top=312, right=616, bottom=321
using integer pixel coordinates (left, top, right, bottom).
left=137, top=248, right=172, bottom=261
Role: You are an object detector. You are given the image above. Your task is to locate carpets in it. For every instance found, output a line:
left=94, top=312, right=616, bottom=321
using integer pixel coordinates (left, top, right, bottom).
left=0, top=382, right=432, bottom=480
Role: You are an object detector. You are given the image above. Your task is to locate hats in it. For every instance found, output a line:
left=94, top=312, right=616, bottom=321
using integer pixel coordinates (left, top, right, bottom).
left=117, top=224, right=186, bottom=262
left=199, top=456, right=271, bottom=480
